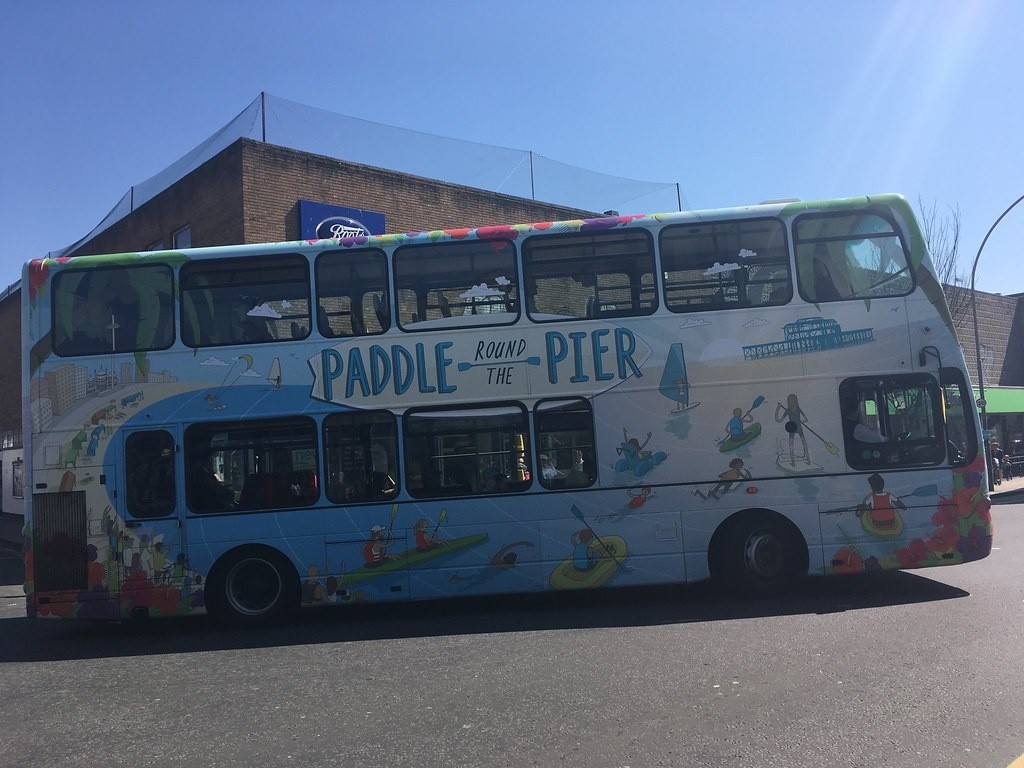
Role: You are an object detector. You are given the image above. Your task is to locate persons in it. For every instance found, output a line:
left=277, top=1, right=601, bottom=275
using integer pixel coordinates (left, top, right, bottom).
left=189, top=443, right=237, bottom=511
left=990, top=437, right=1013, bottom=486
left=847, top=400, right=891, bottom=463
left=575, top=449, right=593, bottom=476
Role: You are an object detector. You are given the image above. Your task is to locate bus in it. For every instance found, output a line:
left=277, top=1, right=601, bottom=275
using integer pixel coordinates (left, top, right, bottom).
left=21, top=193, right=992, bottom=631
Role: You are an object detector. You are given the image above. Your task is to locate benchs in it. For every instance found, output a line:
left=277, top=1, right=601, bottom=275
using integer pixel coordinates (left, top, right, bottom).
left=235, top=300, right=276, bottom=344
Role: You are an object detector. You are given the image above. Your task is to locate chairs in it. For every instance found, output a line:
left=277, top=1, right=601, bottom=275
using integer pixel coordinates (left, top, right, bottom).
left=438, top=290, right=452, bottom=319
left=372, top=294, right=390, bottom=330
left=238, top=471, right=278, bottom=510
left=292, top=324, right=308, bottom=337
left=348, top=313, right=367, bottom=332
left=285, top=470, right=319, bottom=507
left=340, top=463, right=375, bottom=501
left=418, top=457, right=473, bottom=497
left=319, top=305, right=333, bottom=338
left=190, top=468, right=228, bottom=514
left=411, top=314, right=422, bottom=323
